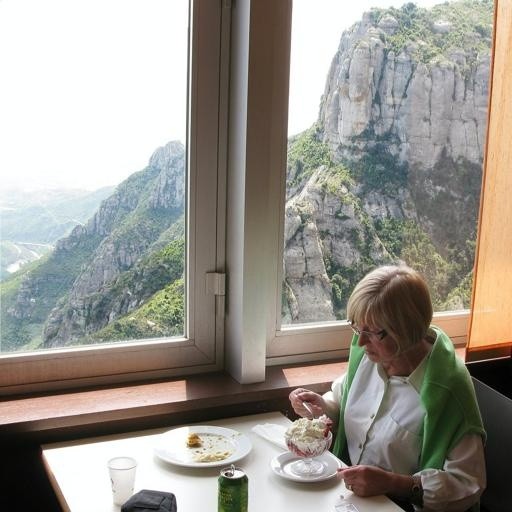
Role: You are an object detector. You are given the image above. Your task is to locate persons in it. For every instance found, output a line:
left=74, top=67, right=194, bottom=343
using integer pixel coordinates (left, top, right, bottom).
left=287, top=266, right=489, bottom=511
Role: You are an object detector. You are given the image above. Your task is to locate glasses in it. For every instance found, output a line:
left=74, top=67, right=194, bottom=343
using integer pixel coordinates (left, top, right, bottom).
left=346, top=318, right=388, bottom=346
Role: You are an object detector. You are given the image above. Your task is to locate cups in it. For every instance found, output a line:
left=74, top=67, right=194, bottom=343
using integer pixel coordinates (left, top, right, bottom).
left=107, top=456, right=137, bottom=507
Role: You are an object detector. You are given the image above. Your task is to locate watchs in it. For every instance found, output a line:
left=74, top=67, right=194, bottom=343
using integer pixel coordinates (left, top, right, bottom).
left=410, top=472, right=421, bottom=498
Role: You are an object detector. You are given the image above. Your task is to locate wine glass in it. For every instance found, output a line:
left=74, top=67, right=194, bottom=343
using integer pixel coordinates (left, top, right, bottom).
left=284, top=431, right=333, bottom=475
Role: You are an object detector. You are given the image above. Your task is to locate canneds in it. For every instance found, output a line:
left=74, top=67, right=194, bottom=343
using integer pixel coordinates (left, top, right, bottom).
left=217, top=464, right=248, bottom=512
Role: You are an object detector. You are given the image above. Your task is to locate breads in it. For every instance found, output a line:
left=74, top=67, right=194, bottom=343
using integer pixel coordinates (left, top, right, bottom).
left=187, top=432, right=200, bottom=446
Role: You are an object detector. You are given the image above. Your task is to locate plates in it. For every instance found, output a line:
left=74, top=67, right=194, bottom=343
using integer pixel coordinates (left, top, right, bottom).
left=270, top=450, right=341, bottom=483
left=156, top=425, right=253, bottom=468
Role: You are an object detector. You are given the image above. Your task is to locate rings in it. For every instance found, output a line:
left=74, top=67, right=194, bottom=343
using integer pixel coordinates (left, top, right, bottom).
left=349, top=484, right=353, bottom=491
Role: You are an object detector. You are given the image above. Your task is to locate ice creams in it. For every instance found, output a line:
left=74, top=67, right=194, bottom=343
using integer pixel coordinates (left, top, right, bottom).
left=285, top=415, right=333, bottom=459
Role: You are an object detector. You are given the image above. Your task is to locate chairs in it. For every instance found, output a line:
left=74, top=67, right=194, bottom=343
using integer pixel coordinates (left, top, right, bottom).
left=468, top=374, right=511, bottom=510
